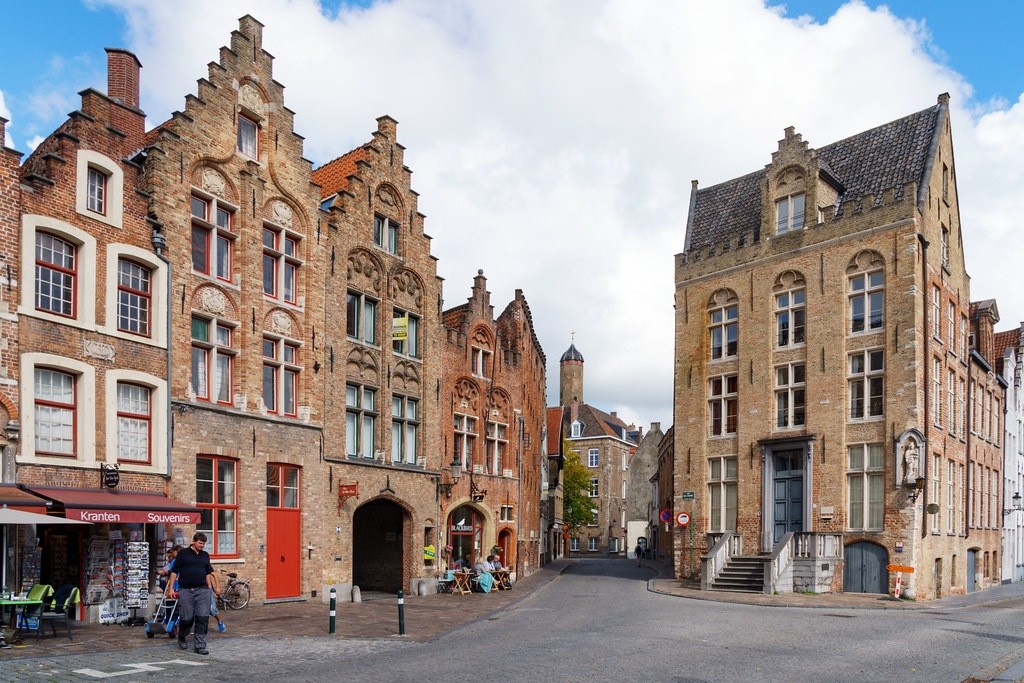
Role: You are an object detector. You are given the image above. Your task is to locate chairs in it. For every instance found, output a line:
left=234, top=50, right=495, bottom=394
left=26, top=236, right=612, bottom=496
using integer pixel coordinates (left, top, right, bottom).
left=17, top=583, right=81, bottom=645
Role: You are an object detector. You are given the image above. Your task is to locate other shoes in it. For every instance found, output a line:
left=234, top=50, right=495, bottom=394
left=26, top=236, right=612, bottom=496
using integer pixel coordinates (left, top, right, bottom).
left=494, top=581, right=500, bottom=587
left=638, top=565, right=642, bottom=567
left=194, top=648, right=209, bottom=655
left=178, top=640, right=187, bottom=649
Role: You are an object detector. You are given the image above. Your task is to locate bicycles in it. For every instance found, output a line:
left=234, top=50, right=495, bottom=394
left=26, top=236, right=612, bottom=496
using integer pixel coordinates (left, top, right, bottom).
left=212, top=569, right=251, bottom=612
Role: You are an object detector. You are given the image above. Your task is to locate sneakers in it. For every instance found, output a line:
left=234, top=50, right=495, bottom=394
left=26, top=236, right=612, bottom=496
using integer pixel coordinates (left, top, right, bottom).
left=218, top=620, right=226, bottom=633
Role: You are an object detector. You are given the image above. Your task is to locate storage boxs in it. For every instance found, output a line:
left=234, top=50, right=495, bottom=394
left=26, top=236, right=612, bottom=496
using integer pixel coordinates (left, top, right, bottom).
left=83, top=530, right=149, bottom=624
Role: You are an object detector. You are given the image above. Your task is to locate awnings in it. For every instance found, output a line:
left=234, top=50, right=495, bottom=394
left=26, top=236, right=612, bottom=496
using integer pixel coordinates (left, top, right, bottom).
left=18, top=483, right=203, bottom=525
left=0, top=483, right=53, bottom=515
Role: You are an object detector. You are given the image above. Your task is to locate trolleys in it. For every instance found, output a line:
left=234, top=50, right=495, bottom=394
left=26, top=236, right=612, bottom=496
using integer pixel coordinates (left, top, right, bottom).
left=145, top=578, right=179, bottom=639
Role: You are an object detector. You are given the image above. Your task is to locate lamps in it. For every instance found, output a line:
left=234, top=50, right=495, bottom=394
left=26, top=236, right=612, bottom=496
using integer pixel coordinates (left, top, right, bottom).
left=1005, top=492, right=1021, bottom=516
left=441, top=485, right=456, bottom=501
left=908, top=476, right=926, bottom=502
left=440, top=455, right=463, bottom=487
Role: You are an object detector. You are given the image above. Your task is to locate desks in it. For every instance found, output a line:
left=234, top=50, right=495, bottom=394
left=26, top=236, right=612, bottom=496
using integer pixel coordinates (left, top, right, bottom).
left=450, top=572, right=474, bottom=596
left=492, top=570, right=513, bottom=591
left=0, top=599, right=42, bottom=645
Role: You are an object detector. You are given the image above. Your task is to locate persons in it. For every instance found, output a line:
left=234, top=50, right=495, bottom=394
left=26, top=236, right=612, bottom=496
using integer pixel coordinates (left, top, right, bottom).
left=634, top=543, right=645, bottom=567
left=157, top=532, right=226, bottom=655
left=449, top=553, right=471, bottom=569
left=472, top=555, right=511, bottom=592
left=904, top=441, right=918, bottom=479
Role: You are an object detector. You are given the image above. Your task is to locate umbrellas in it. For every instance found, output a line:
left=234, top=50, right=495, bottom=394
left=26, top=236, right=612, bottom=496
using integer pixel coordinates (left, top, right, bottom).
left=0, top=508, right=94, bottom=597
